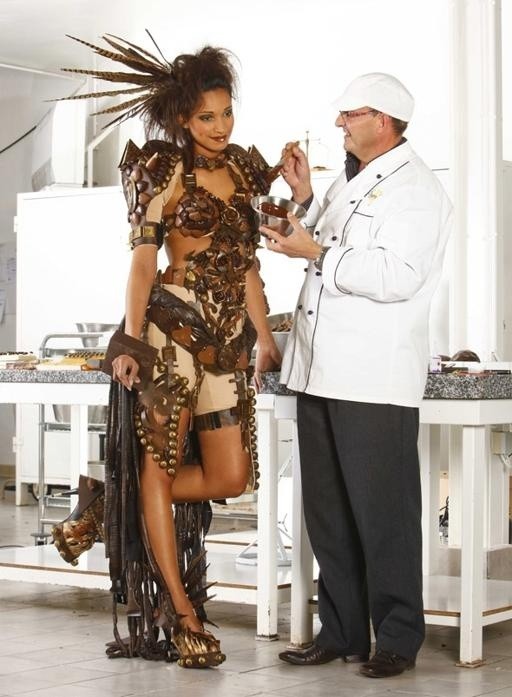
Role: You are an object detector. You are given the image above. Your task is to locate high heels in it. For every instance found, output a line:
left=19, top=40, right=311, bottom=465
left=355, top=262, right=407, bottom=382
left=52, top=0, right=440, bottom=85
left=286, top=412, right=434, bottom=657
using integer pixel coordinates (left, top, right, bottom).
left=156, top=597, right=226, bottom=668
left=51, top=475, right=104, bottom=567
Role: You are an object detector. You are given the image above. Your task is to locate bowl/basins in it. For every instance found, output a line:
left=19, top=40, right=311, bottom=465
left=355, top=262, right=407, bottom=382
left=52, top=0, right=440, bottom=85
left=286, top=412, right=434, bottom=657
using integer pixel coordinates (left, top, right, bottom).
left=249, top=194, right=307, bottom=239
left=76, top=322, right=122, bottom=347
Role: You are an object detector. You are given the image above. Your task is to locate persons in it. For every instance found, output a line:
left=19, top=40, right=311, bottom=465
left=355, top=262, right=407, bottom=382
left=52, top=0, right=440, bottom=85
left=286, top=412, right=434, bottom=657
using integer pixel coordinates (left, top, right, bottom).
left=257, top=71, right=453, bottom=677
left=51, top=45, right=281, bottom=670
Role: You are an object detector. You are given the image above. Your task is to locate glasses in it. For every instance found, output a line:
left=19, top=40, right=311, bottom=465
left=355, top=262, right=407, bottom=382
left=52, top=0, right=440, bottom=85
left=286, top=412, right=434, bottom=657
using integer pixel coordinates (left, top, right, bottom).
left=339, top=108, right=375, bottom=122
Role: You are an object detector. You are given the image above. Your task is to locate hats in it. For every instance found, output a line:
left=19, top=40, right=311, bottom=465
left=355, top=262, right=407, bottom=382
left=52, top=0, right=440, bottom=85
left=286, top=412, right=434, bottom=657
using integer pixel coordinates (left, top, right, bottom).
left=335, top=73, right=415, bottom=123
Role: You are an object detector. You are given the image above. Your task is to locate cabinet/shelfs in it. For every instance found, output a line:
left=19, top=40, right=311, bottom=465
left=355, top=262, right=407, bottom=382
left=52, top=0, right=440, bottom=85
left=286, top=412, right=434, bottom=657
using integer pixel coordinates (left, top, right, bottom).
left=15, top=184, right=128, bottom=511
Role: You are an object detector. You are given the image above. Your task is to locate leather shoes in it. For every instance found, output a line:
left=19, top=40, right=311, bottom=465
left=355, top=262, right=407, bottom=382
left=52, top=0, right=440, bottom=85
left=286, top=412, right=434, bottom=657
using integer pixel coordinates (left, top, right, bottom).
left=279, top=642, right=369, bottom=667
left=360, top=649, right=416, bottom=680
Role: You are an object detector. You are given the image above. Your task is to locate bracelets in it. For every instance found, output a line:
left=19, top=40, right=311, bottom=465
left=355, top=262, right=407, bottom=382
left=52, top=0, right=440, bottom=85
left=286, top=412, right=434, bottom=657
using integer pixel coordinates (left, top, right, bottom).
left=313, top=247, right=324, bottom=267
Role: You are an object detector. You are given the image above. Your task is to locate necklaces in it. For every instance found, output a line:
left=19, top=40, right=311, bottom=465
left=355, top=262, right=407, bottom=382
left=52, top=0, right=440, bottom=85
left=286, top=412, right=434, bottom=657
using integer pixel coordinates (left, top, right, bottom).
left=194, top=151, right=229, bottom=172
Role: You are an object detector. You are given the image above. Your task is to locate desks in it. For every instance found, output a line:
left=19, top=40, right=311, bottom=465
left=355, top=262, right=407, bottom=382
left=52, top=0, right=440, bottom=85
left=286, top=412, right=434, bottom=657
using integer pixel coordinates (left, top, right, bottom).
left=0, top=366, right=512, bottom=668
